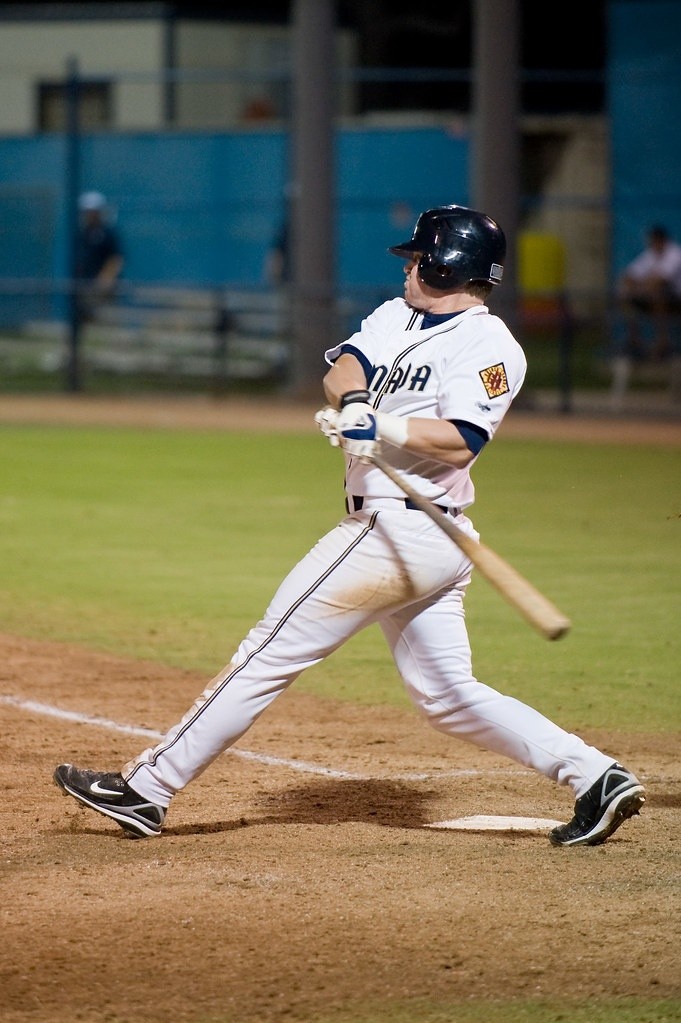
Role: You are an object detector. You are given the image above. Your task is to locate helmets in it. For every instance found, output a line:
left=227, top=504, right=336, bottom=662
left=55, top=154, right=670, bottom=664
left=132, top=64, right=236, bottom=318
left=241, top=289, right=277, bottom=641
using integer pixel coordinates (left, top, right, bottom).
left=389, top=204, right=507, bottom=290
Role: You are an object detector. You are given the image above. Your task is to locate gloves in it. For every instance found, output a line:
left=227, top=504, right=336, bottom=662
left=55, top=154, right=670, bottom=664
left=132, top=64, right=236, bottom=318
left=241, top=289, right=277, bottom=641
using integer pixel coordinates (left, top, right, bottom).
left=314, top=403, right=388, bottom=446
left=336, top=390, right=381, bottom=466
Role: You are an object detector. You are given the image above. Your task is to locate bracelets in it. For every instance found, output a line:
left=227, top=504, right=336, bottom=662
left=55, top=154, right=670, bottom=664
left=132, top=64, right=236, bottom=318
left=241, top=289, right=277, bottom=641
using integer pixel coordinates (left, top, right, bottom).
left=375, top=412, right=408, bottom=449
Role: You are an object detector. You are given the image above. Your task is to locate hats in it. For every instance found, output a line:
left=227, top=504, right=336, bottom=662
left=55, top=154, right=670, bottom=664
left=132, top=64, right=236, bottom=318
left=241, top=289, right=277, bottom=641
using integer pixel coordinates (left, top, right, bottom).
left=79, top=193, right=106, bottom=212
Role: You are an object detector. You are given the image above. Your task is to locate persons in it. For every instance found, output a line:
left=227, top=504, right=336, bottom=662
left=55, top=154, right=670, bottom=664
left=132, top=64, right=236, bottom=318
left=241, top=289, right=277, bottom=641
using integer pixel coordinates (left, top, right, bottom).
left=72, top=192, right=126, bottom=318
left=53, top=205, right=646, bottom=847
left=271, top=191, right=295, bottom=336
left=618, top=225, right=681, bottom=362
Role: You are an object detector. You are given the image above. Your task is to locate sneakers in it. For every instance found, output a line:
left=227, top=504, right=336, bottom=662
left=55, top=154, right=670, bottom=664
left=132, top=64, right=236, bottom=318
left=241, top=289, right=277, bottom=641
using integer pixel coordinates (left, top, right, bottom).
left=548, top=763, right=645, bottom=847
left=53, top=764, right=167, bottom=837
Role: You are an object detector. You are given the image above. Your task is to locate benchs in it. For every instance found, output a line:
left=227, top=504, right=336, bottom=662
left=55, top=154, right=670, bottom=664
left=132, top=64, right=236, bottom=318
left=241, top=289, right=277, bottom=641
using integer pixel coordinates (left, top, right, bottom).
left=0, top=285, right=355, bottom=390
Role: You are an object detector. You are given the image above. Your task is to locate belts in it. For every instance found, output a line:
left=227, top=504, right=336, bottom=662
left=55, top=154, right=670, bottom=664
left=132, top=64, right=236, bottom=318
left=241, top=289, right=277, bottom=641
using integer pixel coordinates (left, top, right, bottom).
left=345, top=496, right=460, bottom=518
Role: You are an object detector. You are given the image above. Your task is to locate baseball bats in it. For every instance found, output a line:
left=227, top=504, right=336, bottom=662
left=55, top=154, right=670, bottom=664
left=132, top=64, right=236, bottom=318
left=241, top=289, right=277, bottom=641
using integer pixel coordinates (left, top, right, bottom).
left=315, top=407, right=572, bottom=642
